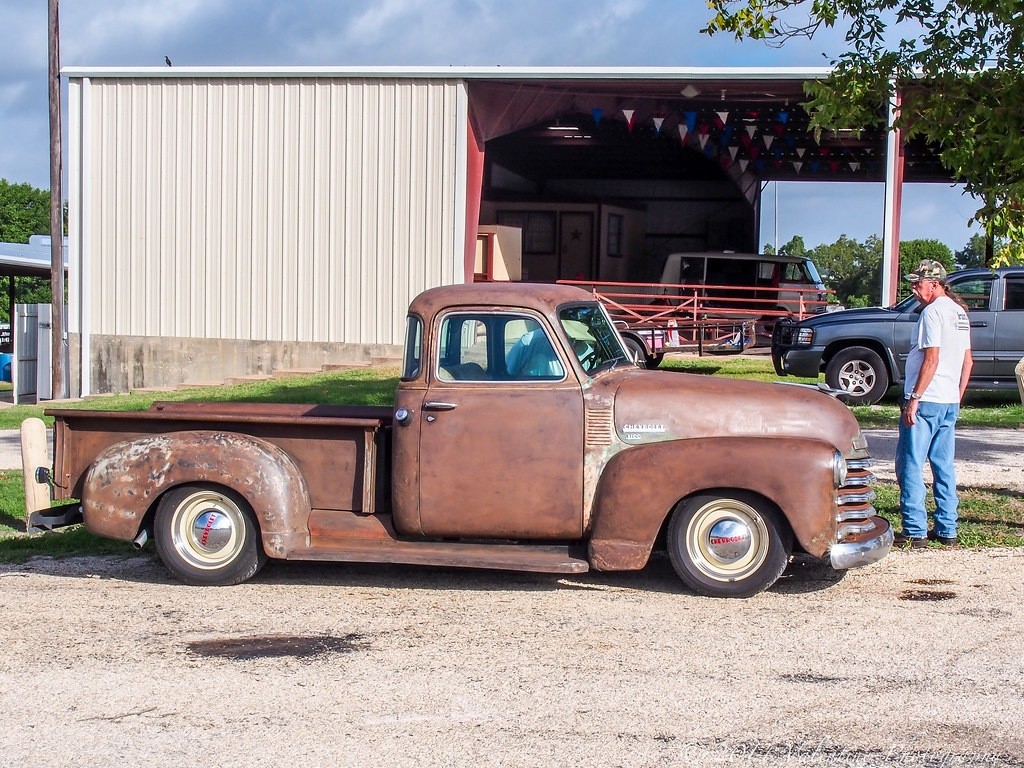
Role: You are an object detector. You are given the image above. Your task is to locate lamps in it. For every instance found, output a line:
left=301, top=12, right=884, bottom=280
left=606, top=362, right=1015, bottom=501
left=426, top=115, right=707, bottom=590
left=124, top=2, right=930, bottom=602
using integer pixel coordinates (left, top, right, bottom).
left=681, top=84, right=702, bottom=97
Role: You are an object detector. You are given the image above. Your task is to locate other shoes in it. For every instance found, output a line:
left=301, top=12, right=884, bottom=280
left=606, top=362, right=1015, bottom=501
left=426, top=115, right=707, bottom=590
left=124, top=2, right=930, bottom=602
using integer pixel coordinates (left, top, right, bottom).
left=927, top=529, right=957, bottom=546
left=893, top=534, right=928, bottom=547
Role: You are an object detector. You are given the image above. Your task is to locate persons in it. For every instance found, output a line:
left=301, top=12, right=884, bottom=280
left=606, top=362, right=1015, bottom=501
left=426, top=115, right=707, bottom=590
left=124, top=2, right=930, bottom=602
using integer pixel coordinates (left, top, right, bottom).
left=892, top=254, right=976, bottom=548
left=504, top=309, right=597, bottom=377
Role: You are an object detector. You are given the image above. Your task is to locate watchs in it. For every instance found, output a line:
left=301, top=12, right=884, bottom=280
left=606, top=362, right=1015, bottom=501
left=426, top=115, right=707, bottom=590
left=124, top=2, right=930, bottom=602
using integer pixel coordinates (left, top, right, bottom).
left=909, top=392, right=922, bottom=400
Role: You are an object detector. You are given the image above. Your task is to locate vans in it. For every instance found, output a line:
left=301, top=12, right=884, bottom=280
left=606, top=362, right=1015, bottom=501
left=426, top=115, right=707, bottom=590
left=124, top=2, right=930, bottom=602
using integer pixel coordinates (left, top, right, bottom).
left=657, top=248, right=829, bottom=313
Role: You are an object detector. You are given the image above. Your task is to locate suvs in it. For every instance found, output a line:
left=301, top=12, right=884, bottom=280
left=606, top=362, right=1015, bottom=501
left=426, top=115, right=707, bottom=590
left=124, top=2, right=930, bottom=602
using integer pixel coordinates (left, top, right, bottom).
left=770, top=267, right=1024, bottom=405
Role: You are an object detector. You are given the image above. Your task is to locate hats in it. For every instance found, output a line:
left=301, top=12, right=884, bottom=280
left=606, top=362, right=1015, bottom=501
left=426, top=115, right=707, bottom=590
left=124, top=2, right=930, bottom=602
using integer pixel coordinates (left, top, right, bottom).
left=902, top=259, right=946, bottom=282
left=561, top=320, right=596, bottom=341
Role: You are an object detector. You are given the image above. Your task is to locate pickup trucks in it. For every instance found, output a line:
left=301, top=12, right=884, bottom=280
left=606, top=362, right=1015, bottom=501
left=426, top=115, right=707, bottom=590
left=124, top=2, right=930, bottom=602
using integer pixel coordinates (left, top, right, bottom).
left=28, top=280, right=895, bottom=598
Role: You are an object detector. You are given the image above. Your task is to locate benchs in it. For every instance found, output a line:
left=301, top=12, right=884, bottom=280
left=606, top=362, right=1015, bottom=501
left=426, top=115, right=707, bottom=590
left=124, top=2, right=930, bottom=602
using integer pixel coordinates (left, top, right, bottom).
left=440, top=362, right=490, bottom=382
